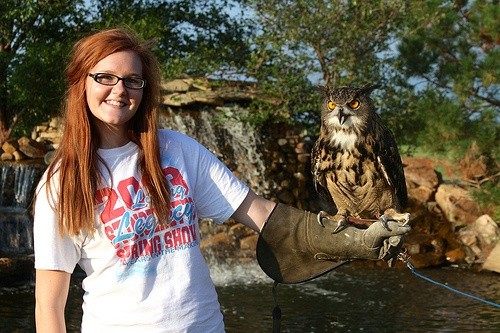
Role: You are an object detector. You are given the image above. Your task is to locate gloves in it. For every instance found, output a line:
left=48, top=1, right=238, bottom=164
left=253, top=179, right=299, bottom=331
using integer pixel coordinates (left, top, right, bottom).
left=255, top=203, right=411, bottom=284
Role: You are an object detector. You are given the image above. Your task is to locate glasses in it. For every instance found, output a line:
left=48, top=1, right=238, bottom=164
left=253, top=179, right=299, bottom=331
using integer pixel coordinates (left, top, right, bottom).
left=87, top=73, right=146, bottom=90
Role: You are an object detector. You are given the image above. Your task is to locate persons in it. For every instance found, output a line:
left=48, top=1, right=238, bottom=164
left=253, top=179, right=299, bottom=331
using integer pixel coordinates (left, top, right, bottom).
left=33, top=28, right=411, bottom=333
